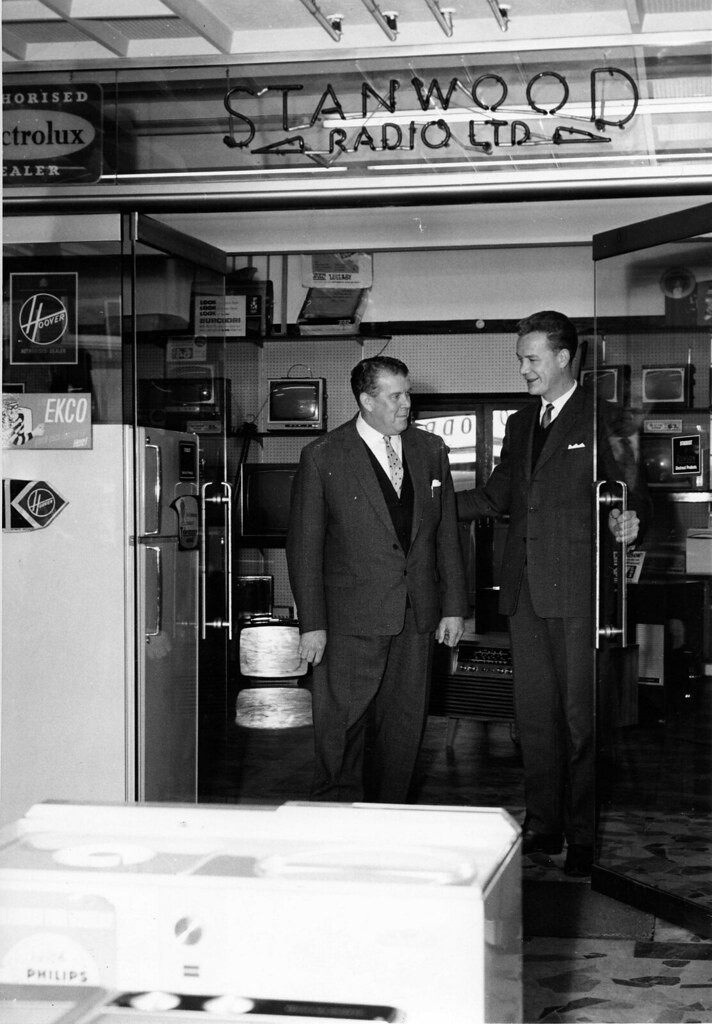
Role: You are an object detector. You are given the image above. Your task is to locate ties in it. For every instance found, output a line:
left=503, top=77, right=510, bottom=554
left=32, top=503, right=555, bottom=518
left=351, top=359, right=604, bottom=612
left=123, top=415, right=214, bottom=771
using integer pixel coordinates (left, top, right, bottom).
left=383, top=436, right=404, bottom=498
left=541, top=403, right=555, bottom=429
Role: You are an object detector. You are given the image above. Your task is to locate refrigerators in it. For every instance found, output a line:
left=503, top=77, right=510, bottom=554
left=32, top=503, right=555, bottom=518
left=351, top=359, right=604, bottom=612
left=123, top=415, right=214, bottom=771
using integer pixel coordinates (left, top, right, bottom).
left=0, top=423, right=199, bottom=830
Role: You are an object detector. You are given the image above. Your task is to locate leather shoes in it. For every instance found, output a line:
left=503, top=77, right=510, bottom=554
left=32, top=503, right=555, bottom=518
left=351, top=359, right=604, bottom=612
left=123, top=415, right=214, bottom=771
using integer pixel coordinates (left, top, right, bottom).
left=566, top=842, right=593, bottom=877
left=520, top=828, right=563, bottom=855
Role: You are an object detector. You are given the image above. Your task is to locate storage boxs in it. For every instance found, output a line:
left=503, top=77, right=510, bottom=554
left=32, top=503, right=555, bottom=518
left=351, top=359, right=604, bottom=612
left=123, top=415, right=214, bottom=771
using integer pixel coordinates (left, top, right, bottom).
left=686, top=529, right=712, bottom=574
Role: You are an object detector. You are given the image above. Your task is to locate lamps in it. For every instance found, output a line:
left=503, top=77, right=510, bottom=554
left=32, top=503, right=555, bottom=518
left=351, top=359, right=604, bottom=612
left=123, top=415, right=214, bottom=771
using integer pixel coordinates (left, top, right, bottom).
left=361, top=0, right=399, bottom=41
left=425, top=0, right=453, bottom=37
left=301, top=0, right=343, bottom=43
left=487, top=0, right=509, bottom=32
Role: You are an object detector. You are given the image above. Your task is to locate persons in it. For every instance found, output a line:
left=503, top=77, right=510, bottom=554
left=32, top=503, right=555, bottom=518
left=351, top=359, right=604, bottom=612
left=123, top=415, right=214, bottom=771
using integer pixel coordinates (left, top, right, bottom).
left=454, top=310, right=643, bottom=879
left=285, top=356, right=469, bottom=803
left=597, top=363, right=686, bottom=732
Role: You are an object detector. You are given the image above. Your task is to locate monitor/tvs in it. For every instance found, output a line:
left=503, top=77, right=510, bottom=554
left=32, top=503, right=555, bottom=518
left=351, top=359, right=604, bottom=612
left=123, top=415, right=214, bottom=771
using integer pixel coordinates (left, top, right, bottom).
left=580, top=364, right=697, bottom=490
left=240, top=461, right=301, bottom=538
left=267, top=378, right=326, bottom=431
